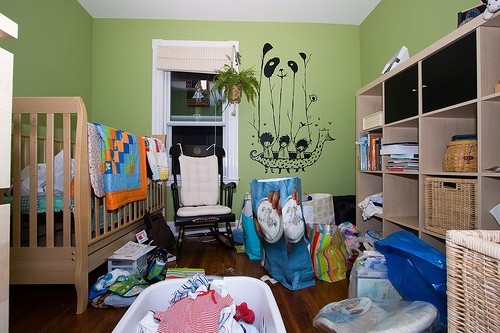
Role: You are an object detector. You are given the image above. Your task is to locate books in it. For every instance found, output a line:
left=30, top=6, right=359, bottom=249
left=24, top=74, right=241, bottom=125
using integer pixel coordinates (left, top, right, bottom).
left=360, top=131, right=382, bottom=171
left=110, top=240, right=157, bottom=260
left=380, top=141, right=419, bottom=172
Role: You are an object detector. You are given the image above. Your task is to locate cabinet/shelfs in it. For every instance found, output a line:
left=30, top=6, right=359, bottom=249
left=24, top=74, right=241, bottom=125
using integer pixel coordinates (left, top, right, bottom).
left=354, top=5, right=500, bottom=257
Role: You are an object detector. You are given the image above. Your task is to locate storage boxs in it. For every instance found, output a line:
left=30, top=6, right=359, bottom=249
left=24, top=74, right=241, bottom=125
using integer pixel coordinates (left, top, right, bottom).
left=355, top=260, right=404, bottom=303
left=362, top=110, right=384, bottom=130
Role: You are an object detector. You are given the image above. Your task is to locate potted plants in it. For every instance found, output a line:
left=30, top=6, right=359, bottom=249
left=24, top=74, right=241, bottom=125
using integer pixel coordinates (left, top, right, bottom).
left=210, top=55, right=261, bottom=114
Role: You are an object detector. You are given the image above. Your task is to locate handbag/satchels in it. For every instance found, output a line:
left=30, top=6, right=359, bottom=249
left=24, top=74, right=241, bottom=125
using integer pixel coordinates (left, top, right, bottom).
left=250, top=177, right=315, bottom=290
left=143, top=249, right=168, bottom=285
left=302, top=193, right=335, bottom=227
left=238, top=193, right=253, bottom=231
left=305, top=222, right=347, bottom=282
left=348, top=252, right=391, bottom=298
left=243, top=211, right=264, bottom=261
left=144, top=213, right=175, bottom=248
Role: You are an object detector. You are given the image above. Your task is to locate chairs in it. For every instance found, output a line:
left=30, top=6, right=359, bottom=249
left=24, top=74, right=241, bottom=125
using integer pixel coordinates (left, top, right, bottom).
left=169, top=144, right=239, bottom=259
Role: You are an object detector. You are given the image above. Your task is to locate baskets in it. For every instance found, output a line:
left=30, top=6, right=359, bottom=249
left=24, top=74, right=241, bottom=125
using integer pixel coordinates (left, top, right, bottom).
left=443, top=140, right=478, bottom=172
left=233, top=229, right=243, bottom=243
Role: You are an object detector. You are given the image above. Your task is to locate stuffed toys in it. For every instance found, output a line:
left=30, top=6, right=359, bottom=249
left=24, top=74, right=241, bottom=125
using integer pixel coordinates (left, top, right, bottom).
left=481, top=0, right=500, bottom=20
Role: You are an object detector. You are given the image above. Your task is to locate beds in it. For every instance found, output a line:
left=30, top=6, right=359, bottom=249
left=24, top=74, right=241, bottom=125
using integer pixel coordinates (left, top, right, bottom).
left=0, top=95, right=167, bottom=315
